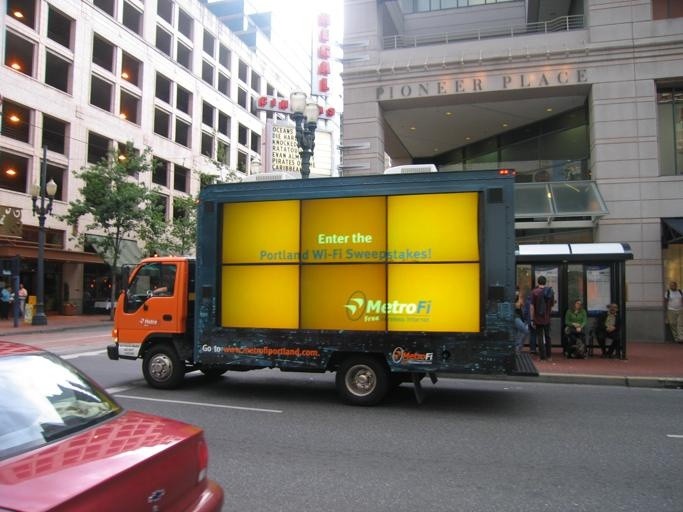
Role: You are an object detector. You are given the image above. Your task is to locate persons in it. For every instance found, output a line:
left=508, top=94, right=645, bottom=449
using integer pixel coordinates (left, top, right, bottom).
left=595, top=302, right=621, bottom=359
left=563, top=297, right=589, bottom=358
left=16, top=283, right=28, bottom=316
left=515, top=287, right=528, bottom=356
left=145, top=270, right=175, bottom=297
left=0, top=286, right=14, bottom=321
left=528, top=275, right=555, bottom=361
left=663, top=281, right=683, bottom=344
left=524, top=289, right=538, bottom=360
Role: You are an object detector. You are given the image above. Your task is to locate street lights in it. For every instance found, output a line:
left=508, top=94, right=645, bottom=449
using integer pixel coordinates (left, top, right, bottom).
left=287, top=88, right=318, bottom=179
left=28, top=176, right=58, bottom=326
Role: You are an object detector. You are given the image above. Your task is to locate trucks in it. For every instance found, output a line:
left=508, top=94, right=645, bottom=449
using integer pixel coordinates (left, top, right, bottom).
left=105, top=168, right=539, bottom=405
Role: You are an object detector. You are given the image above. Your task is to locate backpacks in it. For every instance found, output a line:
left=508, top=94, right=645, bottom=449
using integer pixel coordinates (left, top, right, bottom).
left=570, top=337, right=586, bottom=359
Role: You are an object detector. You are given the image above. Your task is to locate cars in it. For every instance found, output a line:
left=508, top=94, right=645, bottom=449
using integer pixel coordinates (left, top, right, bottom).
left=0, top=340, right=225, bottom=512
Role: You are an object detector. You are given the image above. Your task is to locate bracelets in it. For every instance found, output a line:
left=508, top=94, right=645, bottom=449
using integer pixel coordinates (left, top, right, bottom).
left=530, top=319, right=534, bottom=322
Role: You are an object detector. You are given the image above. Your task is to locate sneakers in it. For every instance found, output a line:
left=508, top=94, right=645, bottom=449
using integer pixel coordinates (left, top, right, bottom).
left=547, top=355, right=552, bottom=362
left=539, top=356, right=545, bottom=363
